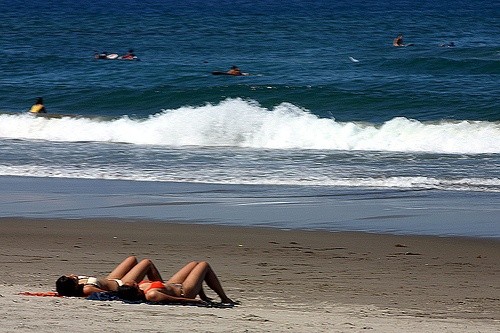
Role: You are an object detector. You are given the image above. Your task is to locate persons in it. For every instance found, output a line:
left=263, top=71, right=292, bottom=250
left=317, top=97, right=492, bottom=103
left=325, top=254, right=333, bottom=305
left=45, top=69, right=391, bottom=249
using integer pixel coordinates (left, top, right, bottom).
left=29, top=97, right=45, bottom=114
left=226, top=65, right=240, bottom=75
left=96, top=51, right=108, bottom=59
left=55, top=256, right=162, bottom=297
left=393, top=33, right=404, bottom=46
left=127, top=49, right=139, bottom=60
left=118, top=260, right=240, bottom=305
